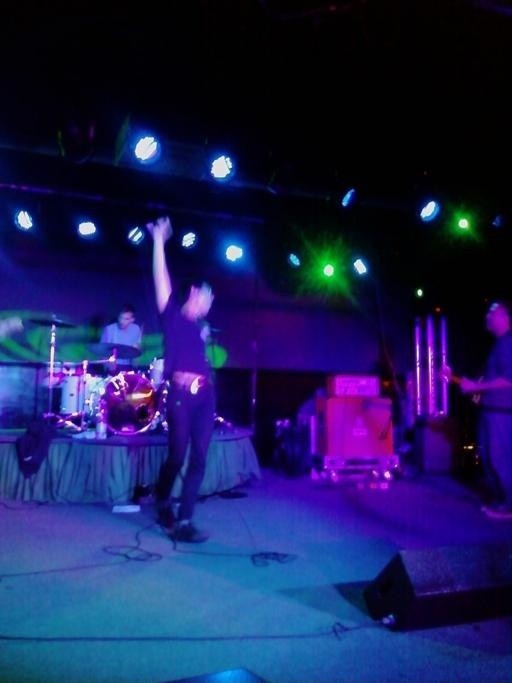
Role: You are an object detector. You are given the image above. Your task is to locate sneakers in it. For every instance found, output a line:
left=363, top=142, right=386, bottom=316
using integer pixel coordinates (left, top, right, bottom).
left=156, top=499, right=177, bottom=528
left=168, top=521, right=210, bottom=543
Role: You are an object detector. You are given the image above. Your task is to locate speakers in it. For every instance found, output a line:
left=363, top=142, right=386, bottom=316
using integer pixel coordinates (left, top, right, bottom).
left=363, top=542, right=512, bottom=631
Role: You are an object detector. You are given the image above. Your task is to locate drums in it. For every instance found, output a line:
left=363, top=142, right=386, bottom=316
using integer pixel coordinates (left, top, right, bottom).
left=147, top=357, right=164, bottom=390
left=42, top=362, right=84, bottom=389
left=59, top=372, right=104, bottom=419
left=89, top=370, right=165, bottom=435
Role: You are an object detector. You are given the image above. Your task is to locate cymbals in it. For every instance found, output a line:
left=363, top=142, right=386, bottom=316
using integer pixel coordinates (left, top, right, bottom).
left=86, top=342, right=142, bottom=358
left=29, top=318, right=77, bottom=328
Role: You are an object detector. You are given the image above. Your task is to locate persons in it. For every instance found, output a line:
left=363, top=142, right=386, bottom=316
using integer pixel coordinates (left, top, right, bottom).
left=460, top=299, right=512, bottom=520
left=147, top=216, right=217, bottom=543
left=100, top=304, right=142, bottom=349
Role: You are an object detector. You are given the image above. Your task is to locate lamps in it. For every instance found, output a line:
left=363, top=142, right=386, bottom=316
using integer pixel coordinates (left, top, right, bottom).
left=289, top=251, right=304, bottom=269
left=353, top=255, right=370, bottom=280
left=14, top=207, right=40, bottom=235
left=176, top=228, right=197, bottom=250
left=202, top=150, right=239, bottom=182
left=338, top=185, right=356, bottom=208
left=71, top=215, right=102, bottom=240
left=123, top=221, right=148, bottom=249
left=411, top=194, right=441, bottom=223
left=220, top=241, right=246, bottom=265
left=123, top=126, right=166, bottom=166
